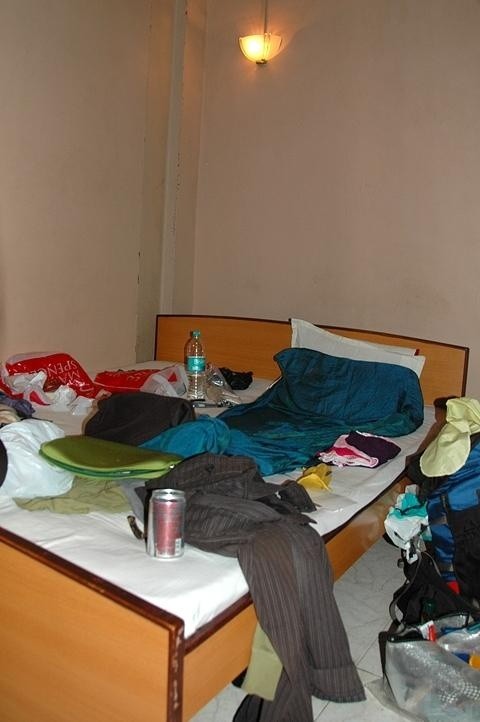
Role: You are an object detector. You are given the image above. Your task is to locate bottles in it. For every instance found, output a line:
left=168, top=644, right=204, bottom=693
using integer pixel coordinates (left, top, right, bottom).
left=185, top=330, right=207, bottom=401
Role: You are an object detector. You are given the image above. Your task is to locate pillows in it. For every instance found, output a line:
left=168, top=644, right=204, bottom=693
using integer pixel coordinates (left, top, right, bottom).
left=267, top=317, right=426, bottom=391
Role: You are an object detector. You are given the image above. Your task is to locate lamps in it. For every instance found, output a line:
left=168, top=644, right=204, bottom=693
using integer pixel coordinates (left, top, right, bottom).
left=238, top=33, right=282, bottom=64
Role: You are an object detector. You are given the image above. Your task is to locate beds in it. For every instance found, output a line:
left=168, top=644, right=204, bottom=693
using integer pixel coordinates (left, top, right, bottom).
left=0, top=315, right=470, bottom=722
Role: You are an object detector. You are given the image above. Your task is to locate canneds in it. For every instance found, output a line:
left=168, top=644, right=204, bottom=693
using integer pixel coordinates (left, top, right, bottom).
left=145, top=488, right=186, bottom=558
